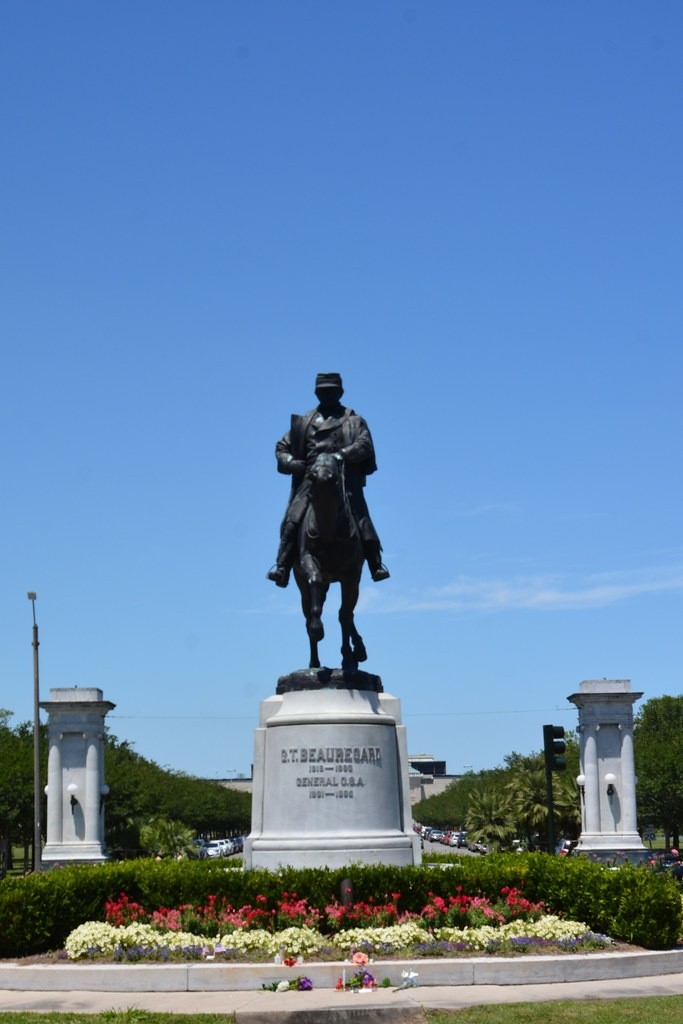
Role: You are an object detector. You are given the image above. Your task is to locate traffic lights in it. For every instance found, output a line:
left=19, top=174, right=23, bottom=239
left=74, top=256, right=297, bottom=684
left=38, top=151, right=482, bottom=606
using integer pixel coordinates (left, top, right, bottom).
left=543, top=721, right=568, bottom=774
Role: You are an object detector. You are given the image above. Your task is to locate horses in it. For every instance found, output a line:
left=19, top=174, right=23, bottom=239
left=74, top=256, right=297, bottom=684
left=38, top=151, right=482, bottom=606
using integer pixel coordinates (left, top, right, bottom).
left=291, top=451, right=367, bottom=671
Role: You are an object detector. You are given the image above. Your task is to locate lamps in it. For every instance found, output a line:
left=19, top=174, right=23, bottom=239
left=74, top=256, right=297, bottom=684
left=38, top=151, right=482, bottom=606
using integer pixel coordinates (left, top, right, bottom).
left=605, top=773, right=616, bottom=804
left=576, top=775, right=585, bottom=805
left=67, top=784, right=78, bottom=815
left=99, top=785, right=110, bottom=815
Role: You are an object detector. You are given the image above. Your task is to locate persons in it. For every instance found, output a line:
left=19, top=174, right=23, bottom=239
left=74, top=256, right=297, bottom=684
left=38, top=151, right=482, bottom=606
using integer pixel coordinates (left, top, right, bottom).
left=266, top=373, right=390, bottom=588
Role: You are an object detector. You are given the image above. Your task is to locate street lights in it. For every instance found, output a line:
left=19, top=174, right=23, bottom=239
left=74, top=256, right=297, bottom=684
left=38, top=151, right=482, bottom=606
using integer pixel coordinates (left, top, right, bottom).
left=28, top=590, right=41, bottom=873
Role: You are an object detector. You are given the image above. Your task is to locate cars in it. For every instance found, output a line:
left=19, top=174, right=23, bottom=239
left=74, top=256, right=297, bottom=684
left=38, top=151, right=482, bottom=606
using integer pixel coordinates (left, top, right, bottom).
left=188, top=836, right=244, bottom=861
left=412, top=825, right=524, bottom=855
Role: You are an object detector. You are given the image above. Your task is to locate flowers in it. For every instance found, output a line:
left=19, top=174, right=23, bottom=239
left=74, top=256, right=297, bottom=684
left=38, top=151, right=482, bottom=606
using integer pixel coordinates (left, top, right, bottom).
left=337, top=969, right=374, bottom=990
left=392, top=970, right=418, bottom=992
left=261, top=976, right=312, bottom=992
